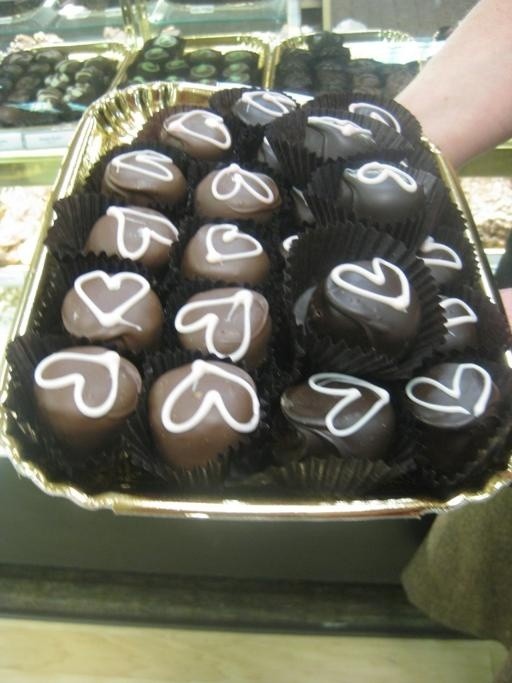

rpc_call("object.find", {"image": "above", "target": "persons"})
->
[392,0,512,353]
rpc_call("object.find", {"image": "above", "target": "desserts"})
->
[1,29,503,471]
